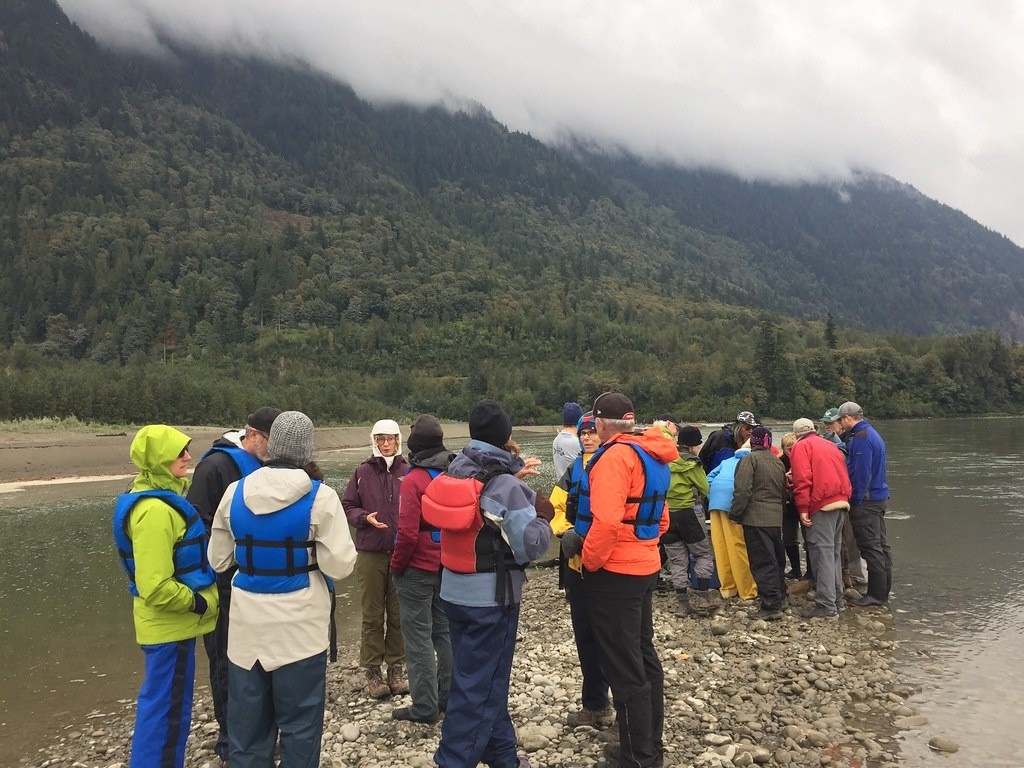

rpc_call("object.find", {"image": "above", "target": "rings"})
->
[802,520,807,524]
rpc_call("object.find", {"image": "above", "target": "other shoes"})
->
[660,562,896,621]
[593,742,663,768]
[393,707,439,724]
[516,757,530,768]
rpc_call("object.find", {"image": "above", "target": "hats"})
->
[593,392,635,420]
[735,411,760,426]
[793,418,816,435]
[407,414,444,452]
[819,407,841,423]
[830,401,863,421]
[749,428,772,448]
[676,426,703,447]
[562,402,583,425]
[657,413,685,428]
[267,411,314,466]
[578,423,596,432]
[246,405,283,434]
[469,398,512,447]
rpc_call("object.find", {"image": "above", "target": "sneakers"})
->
[365,664,412,697]
[567,706,616,727]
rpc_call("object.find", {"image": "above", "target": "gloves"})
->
[190,592,208,614]
[562,529,583,558]
[535,489,555,524]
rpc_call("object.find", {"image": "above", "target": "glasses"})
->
[178,446,188,459]
[375,435,396,445]
[741,425,756,432]
[578,430,597,436]
[822,421,839,426]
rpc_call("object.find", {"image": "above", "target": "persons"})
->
[434,397,556,768]
[184,406,286,761]
[390,413,458,725]
[654,401,893,620]
[343,418,411,699]
[581,391,679,768]
[551,401,584,600]
[204,410,358,768]
[115,424,220,768]
[549,410,621,731]
